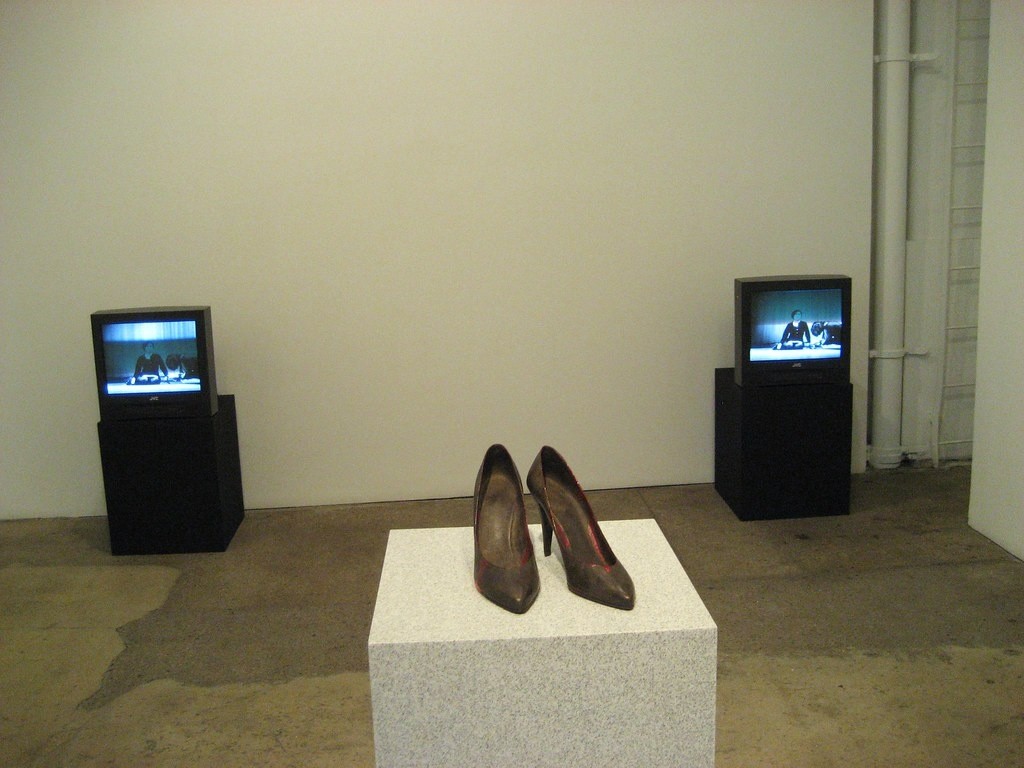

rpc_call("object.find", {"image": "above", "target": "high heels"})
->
[527,445,636,611]
[472,444,541,616]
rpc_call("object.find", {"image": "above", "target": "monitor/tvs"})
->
[90,306,218,421]
[735,274,853,386]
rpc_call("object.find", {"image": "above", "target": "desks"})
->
[96,393,244,557]
[713,367,853,522]
[366,518,719,768]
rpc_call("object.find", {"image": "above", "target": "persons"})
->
[127,342,200,385]
[773,310,842,350]
